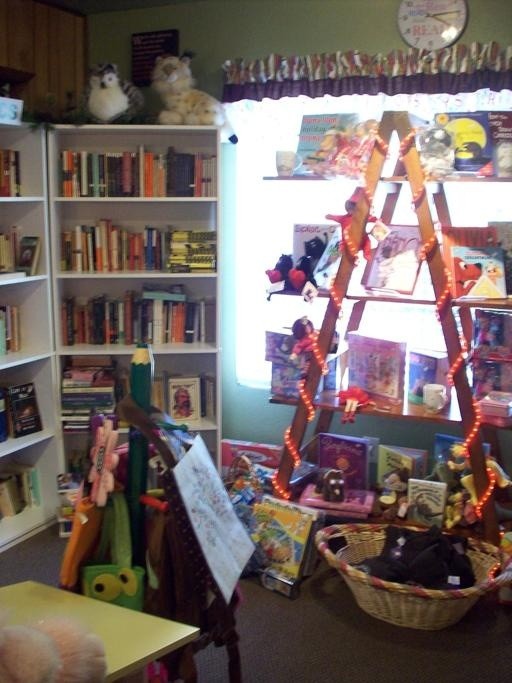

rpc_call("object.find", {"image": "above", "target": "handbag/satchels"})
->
[115,397,256,621]
[81,490,145,607]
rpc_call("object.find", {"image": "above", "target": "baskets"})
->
[315,522,512,633]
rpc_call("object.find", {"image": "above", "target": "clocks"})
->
[393,0,470,52]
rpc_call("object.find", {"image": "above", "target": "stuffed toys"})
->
[150,48,227,126]
[77,60,145,125]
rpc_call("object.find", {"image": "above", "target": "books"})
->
[220,108,512,583]
[55,143,217,538]
[1,149,43,516]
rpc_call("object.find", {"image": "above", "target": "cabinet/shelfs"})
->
[260,163,511,536]
